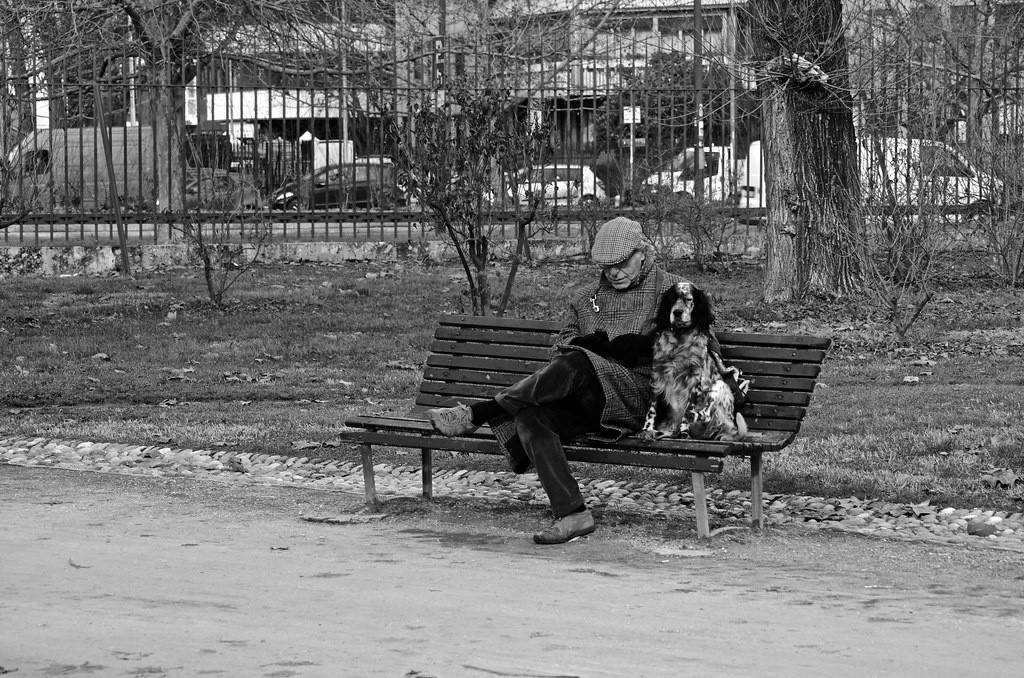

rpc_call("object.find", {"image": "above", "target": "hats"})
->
[591,217,643,266]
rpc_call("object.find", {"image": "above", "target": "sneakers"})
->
[428,401,480,437]
[533,508,596,544]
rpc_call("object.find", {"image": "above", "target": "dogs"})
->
[638,281,751,444]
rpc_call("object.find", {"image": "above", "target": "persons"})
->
[426,217,697,547]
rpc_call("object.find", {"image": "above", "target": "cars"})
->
[482,162,607,210]
[186,174,263,213]
[268,161,407,211]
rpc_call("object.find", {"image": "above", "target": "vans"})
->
[0,125,155,209]
[643,132,1005,209]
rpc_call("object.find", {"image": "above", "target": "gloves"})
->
[610,332,652,366]
[569,331,609,359]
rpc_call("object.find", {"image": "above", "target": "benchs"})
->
[339,313,832,542]
